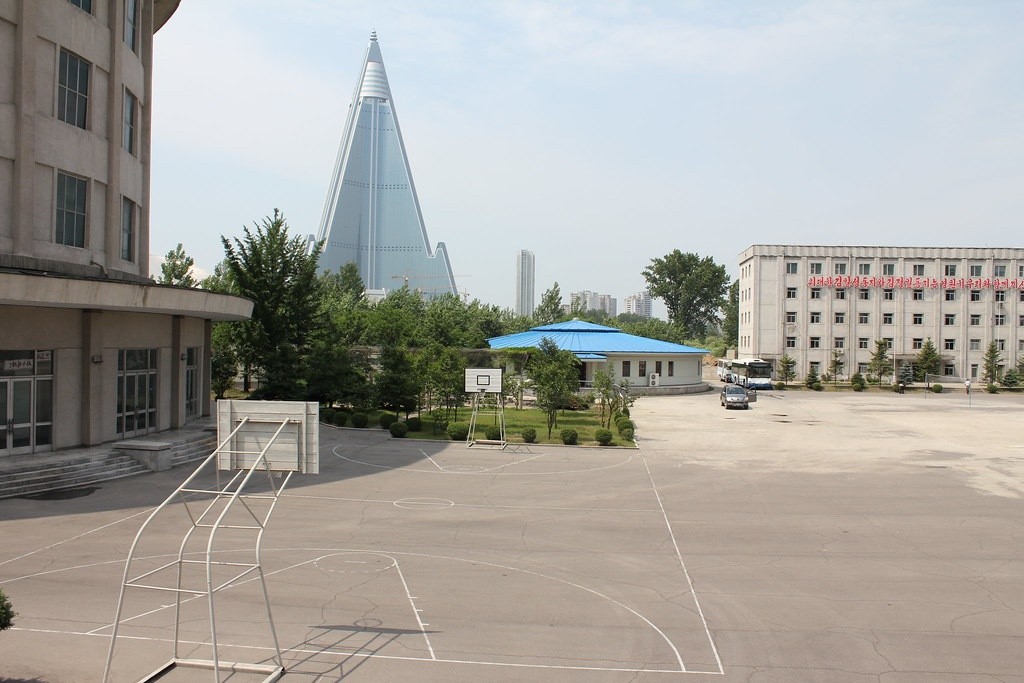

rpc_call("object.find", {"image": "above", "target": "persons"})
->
[898,383,904,394]
[965,379,971,394]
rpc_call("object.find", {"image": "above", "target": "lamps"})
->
[92,354,104,364]
[181,353,189,361]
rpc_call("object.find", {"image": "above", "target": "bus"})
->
[718,358,734,383]
[731,358,774,390]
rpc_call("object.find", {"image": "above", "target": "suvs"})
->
[720,384,757,410]
